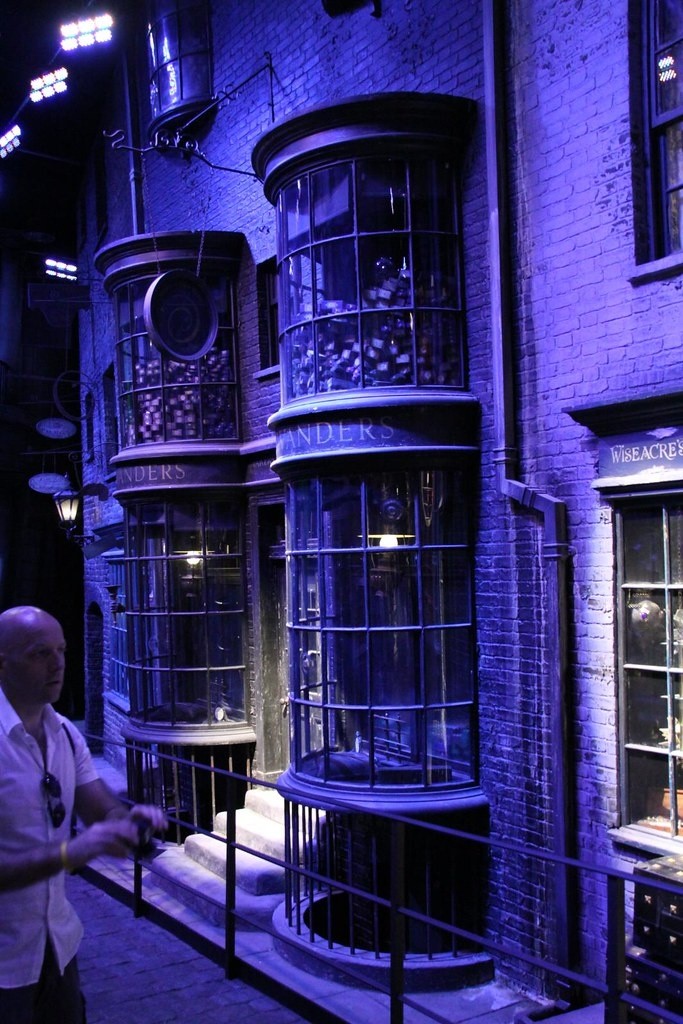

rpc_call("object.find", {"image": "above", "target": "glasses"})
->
[42,770,66,828]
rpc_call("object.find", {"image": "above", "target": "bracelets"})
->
[60,842,70,867]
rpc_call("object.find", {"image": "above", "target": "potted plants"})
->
[647,715,683,821]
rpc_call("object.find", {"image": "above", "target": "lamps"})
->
[173,534,215,596]
[357,473,415,596]
[55,475,95,546]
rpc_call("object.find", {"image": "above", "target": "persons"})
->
[0,606,169,1024]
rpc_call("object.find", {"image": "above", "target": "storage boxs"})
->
[623,927,683,1024]
[633,854,683,966]
